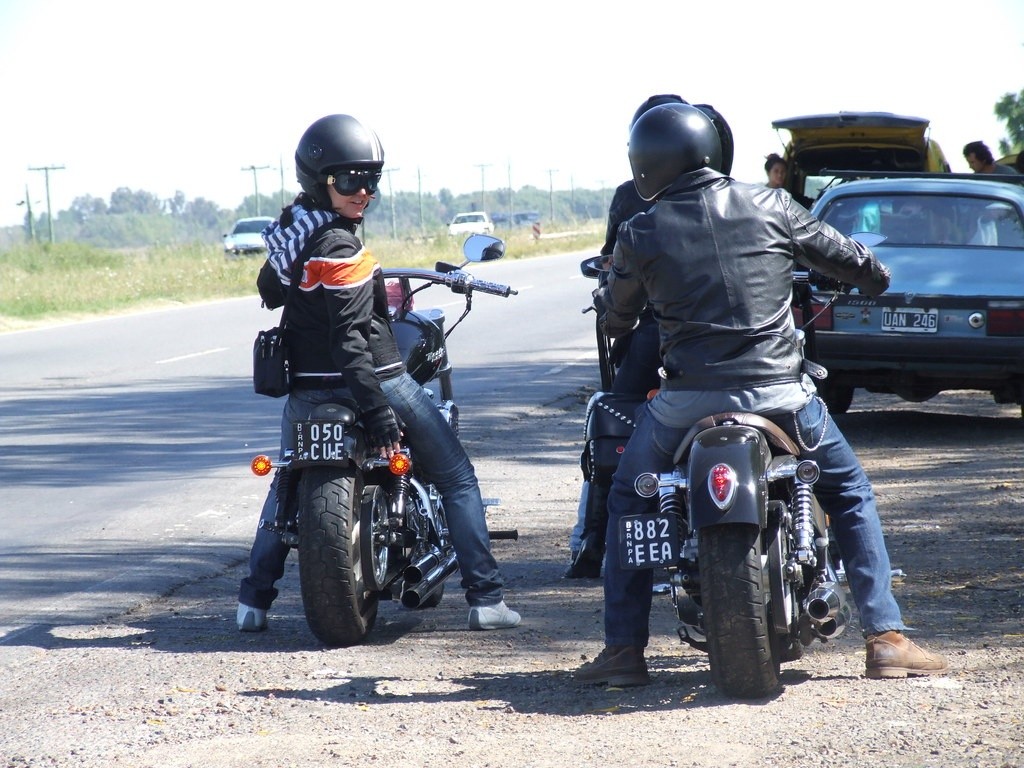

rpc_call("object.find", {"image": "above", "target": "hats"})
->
[629,94,692,135]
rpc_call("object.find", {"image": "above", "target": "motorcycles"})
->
[579,253,858,699]
[253,231,521,647]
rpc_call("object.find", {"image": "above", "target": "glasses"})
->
[331,171,382,197]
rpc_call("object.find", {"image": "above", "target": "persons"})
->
[236,115,522,632]
[565,92,947,688]
[963,141,1024,175]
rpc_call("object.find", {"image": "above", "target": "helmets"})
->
[294,113,383,211]
[691,104,734,176]
[627,103,723,202]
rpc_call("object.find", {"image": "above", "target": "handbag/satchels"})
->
[253,326,294,398]
[583,392,650,481]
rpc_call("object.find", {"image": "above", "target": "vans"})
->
[772,113,957,293]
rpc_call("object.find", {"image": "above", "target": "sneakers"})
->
[467,599,522,631]
[864,629,951,678]
[236,602,267,633]
[572,643,651,687]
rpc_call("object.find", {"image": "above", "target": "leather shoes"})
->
[563,560,602,579]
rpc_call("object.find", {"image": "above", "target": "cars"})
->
[793,170,1024,409]
[446,213,494,236]
[224,217,275,256]
